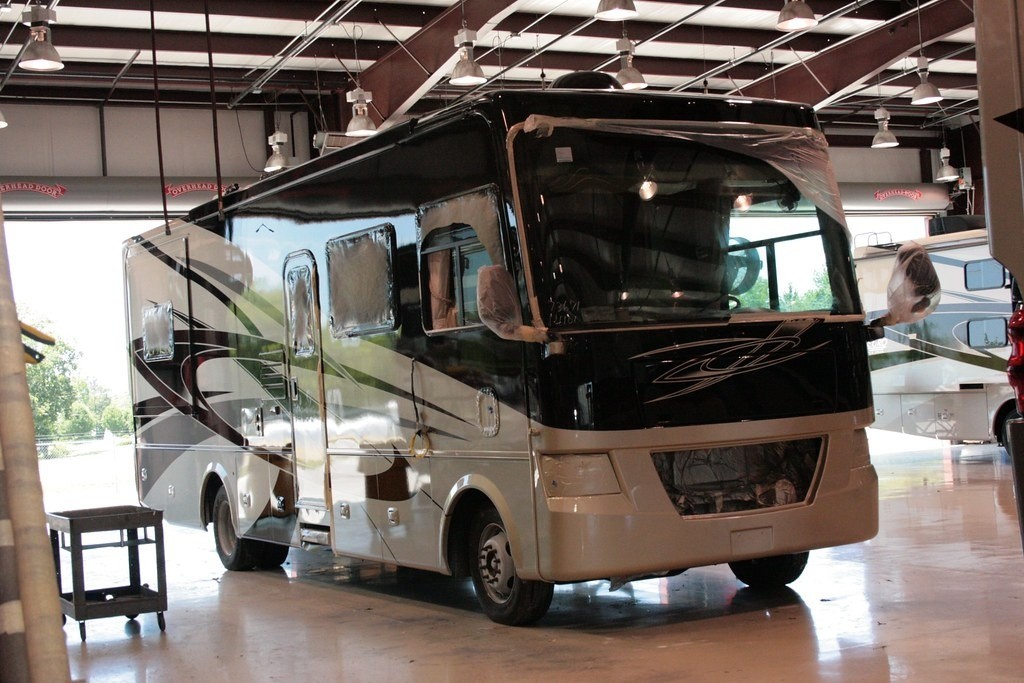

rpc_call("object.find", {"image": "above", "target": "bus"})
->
[118,70,943,627]
[850,213,1024,376]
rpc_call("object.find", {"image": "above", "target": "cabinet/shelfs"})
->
[47,506,168,642]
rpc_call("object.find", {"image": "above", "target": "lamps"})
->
[595,0,640,22]
[449,0,488,86]
[342,22,378,137]
[936,121,959,181]
[776,0,817,31]
[870,73,900,149]
[263,77,288,172]
[910,1,943,104]
[616,20,647,90]
[19,0,64,72]
